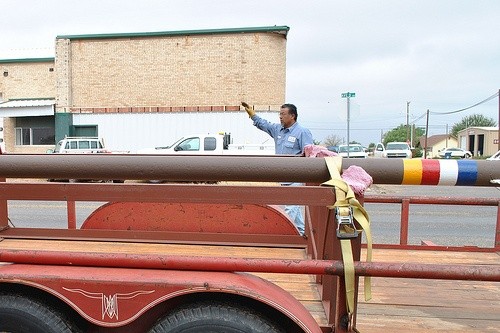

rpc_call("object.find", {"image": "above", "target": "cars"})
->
[436,146,473,160]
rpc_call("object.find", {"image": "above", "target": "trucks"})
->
[0,149,500,333]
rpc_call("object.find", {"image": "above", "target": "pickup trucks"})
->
[123,128,276,186]
[43,135,131,185]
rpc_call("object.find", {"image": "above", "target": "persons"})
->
[245,104,312,235]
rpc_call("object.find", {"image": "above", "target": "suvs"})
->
[334,143,370,159]
[374,141,413,159]
